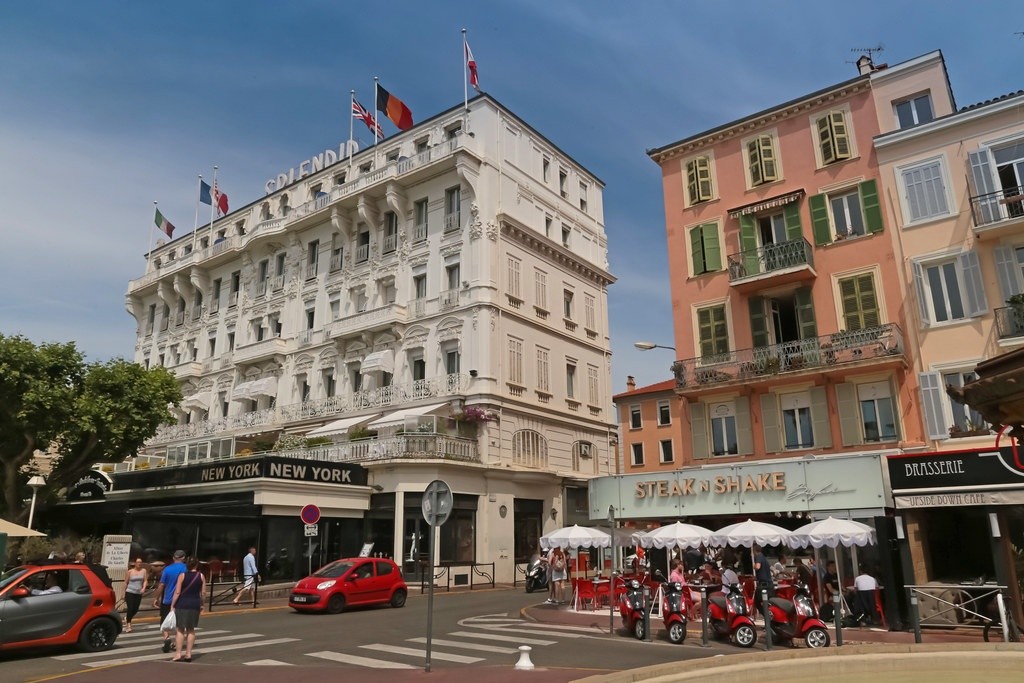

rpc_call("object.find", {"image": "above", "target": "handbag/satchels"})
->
[553,560,565,571]
[819,603,835,622]
[160,611,176,633]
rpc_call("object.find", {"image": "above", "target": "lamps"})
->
[634,342,675,351]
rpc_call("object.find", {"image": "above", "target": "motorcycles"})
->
[524,553,549,593]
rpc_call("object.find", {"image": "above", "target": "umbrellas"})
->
[540,516,877,607]
[0,519,47,536]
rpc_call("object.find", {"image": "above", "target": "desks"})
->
[198,561,231,578]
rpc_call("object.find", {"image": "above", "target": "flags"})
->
[466,39,483,96]
[154,170,229,239]
[351,83,415,139]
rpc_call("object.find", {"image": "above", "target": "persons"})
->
[542,546,879,626]
[76,548,262,662]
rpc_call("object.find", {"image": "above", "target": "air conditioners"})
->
[580,444,589,455]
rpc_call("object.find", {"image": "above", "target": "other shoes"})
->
[233,600,242,606]
[126,626,132,633]
[163,639,171,653]
[561,601,566,605]
[544,598,553,605]
[252,601,259,605]
[553,600,559,605]
[169,643,177,651]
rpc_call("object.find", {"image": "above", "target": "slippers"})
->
[171,657,183,662]
[181,656,191,662]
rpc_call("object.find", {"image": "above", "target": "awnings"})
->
[167,349,396,414]
[306,402,450,439]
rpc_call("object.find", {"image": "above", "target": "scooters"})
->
[707,568,758,648]
[760,584,830,649]
[654,569,693,644]
[611,571,651,641]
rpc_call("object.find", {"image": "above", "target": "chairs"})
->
[208,559,242,582]
[570,572,796,622]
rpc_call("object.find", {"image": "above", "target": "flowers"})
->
[463,407,487,422]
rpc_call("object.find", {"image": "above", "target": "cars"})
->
[0,559,122,654]
[288,557,408,615]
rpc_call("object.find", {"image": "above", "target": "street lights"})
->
[24,475,46,556]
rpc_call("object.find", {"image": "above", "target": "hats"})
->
[174,550,186,559]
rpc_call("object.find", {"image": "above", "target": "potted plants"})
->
[948,416,990,438]
